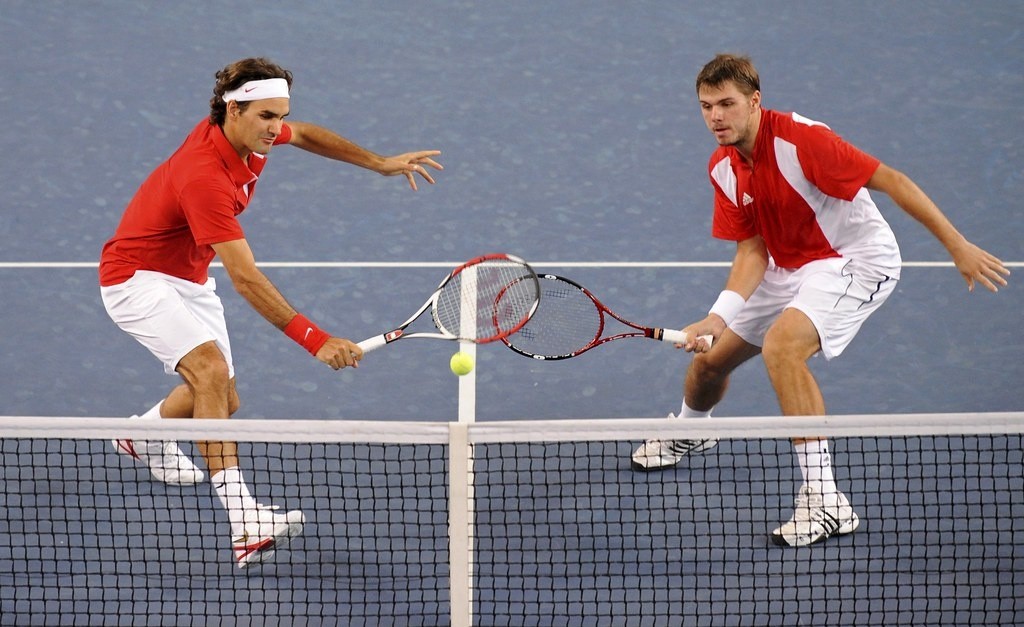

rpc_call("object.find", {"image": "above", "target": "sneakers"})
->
[770,486,860,547]
[231,505,306,568]
[111,415,205,486]
[630,413,720,472]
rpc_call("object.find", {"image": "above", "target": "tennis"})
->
[450,352,474,376]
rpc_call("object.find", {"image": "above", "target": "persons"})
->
[99,56,444,570]
[629,54,1009,547]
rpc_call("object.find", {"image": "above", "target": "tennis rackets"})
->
[490,273,714,361]
[326,253,542,368]
